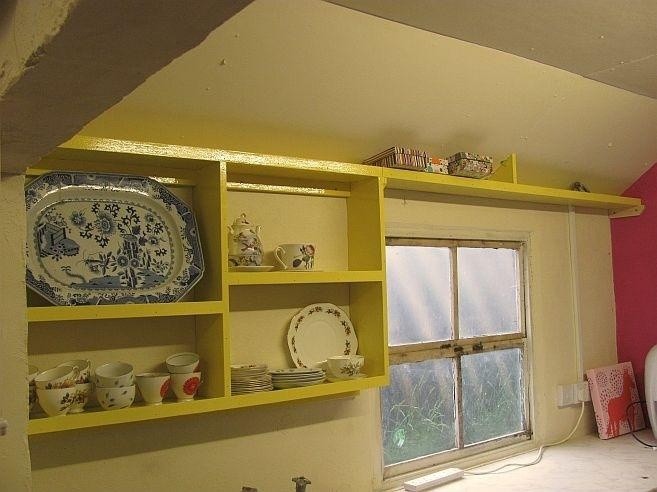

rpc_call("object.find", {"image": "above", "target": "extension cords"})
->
[403,467,464,492]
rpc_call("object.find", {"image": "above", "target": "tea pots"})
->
[228,213,264,266]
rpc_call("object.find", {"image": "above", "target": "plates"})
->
[227,265,273,272]
[277,267,323,271]
[25,172,204,308]
[231,301,358,394]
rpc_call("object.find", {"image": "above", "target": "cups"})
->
[275,242,316,270]
[27,351,204,417]
[328,355,364,379]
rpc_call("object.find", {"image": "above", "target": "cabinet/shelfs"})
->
[25,137,390,434]
